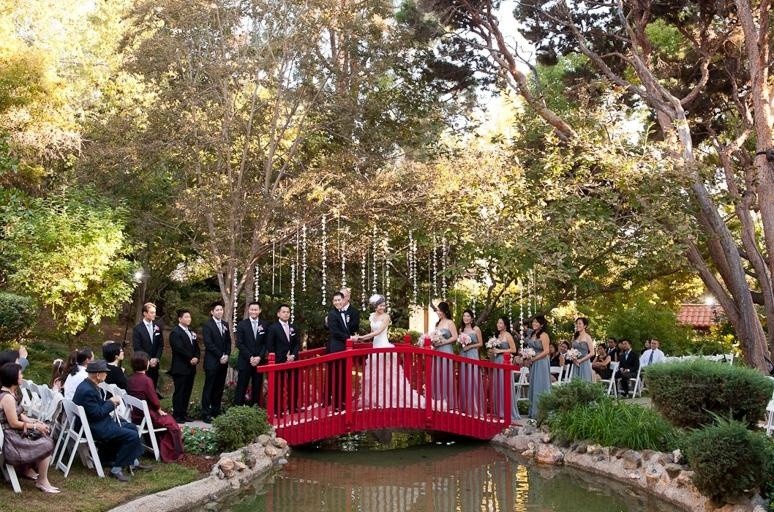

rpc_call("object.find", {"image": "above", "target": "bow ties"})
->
[339,309,346,314]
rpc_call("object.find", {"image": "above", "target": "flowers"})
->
[417,330,443,352]
[457,330,472,349]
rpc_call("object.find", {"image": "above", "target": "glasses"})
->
[120,349,125,352]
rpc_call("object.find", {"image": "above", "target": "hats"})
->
[84,359,113,374]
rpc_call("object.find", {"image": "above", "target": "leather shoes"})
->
[289,406,306,414]
[203,415,215,424]
[276,413,283,419]
[174,415,194,424]
[133,463,155,473]
[109,470,130,482]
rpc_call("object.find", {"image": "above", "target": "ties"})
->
[186,327,193,345]
[625,351,629,361]
[252,320,258,340]
[648,349,655,366]
[217,321,223,336]
[283,323,291,342]
[147,323,154,344]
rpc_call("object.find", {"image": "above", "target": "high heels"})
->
[35,482,61,494]
[23,471,41,481]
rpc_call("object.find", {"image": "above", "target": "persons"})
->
[328,292,358,412]
[458,309,486,418]
[357,294,446,411]
[487,317,522,418]
[325,288,359,408]
[513,316,666,419]
[425,301,458,411]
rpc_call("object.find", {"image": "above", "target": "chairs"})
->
[484,321,774,446]
[0,375,168,496]
